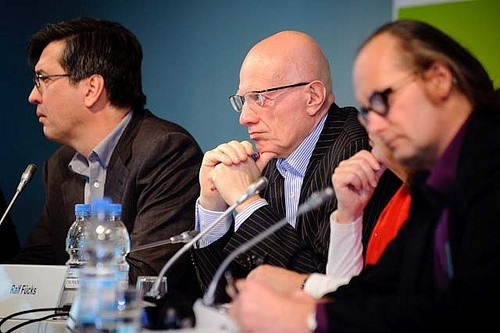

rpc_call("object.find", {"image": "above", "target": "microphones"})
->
[0,163,36,226]
[193,187,334,333]
[148,177,268,299]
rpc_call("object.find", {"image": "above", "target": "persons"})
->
[190,28,374,303]
[229,18,500,333]
[246,130,412,298]
[9,17,204,284]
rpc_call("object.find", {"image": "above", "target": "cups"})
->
[135,276,167,299]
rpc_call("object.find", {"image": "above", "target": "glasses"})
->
[228,82,311,113]
[32,72,72,88]
[355,67,425,125]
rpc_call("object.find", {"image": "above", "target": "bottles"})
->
[80,199,113,324]
[95,204,130,330]
[64,204,93,289]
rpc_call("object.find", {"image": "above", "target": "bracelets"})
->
[300,272,312,291]
[307,310,318,332]
